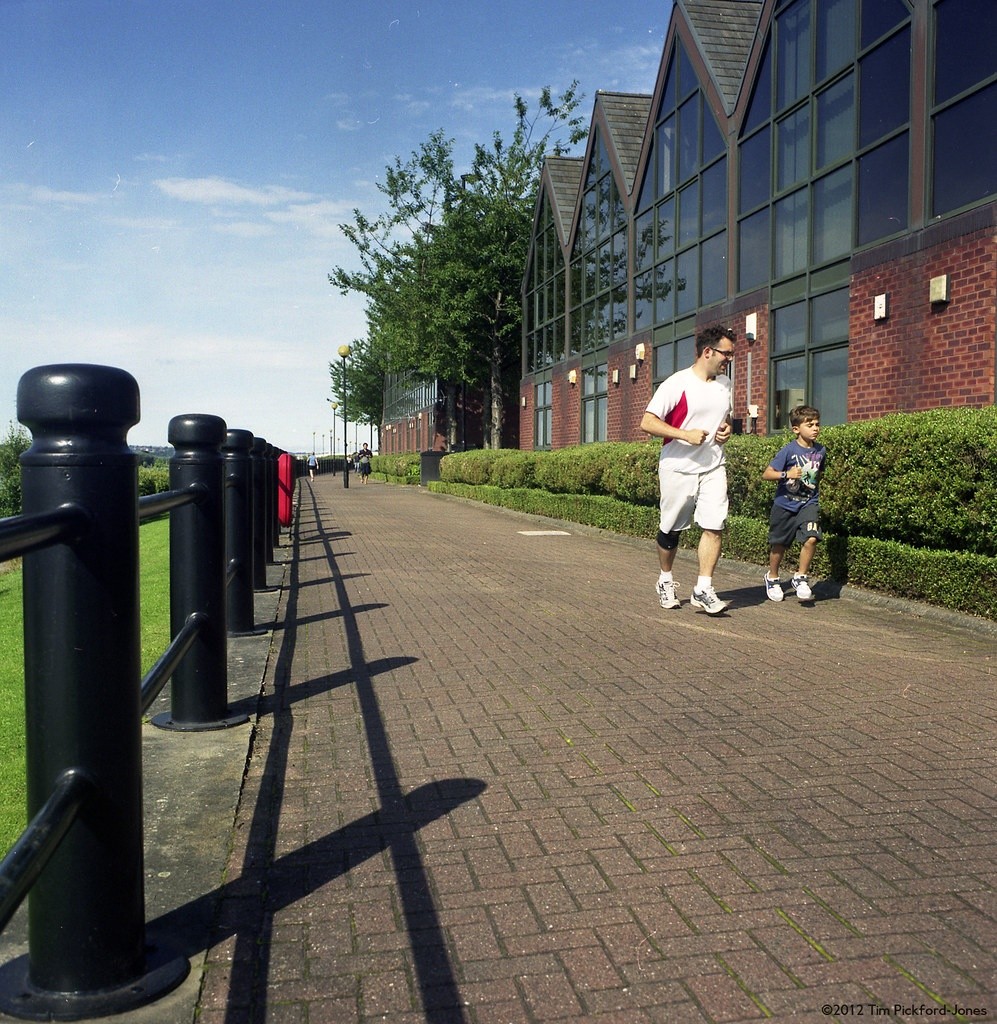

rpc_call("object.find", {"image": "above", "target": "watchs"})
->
[781,471,786,480]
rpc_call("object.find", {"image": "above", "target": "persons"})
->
[762,405,827,603]
[640,325,735,614]
[306,452,319,482]
[359,443,374,485]
[347,451,360,473]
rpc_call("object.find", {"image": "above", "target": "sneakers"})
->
[655,575,682,609]
[791,572,811,599]
[764,570,783,602]
[690,586,728,613]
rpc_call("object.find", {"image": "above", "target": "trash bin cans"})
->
[420,451,449,486]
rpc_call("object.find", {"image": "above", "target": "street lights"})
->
[330,403,337,476]
[337,344,352,488]
[312,429,362,455]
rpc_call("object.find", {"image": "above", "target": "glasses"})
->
[709,347,734,359]
